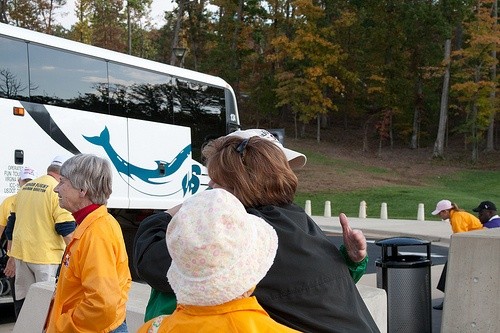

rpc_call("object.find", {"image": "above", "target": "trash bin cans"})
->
[374,238,434,333]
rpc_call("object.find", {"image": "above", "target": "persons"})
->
[145,129,368,323]
[133,136,381,333]
[433,200,500,310]
[5,157,76,283]
[135,188,303,333]
[46,154,132,333]
[0,166,36,322]
[431,199,484,232]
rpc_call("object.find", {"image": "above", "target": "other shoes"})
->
[433,302,443,310]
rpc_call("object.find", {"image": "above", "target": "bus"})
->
[0,22,240,209]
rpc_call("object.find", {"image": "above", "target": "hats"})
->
[166,188,279,307]
[473,201,497,212]
[431,199,455,215]
[51,155,68,167]
[226,128,307,169]
[19,168,36,180]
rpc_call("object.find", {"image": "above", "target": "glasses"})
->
[237,135,260,156]
